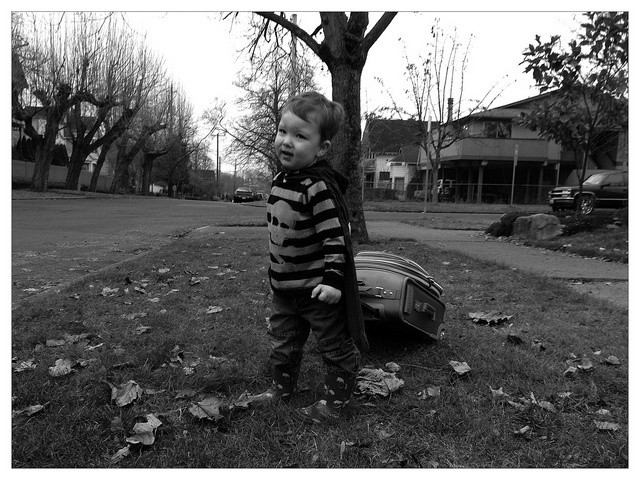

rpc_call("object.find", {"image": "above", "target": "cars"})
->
[235,187,253,200]
[550,171,627,212]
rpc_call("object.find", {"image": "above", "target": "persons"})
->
[254,91,362,424]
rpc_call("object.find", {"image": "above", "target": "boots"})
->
[291,377,356,426]
[246,368,297,408]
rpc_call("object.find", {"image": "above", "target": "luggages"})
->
[354,250,448,347]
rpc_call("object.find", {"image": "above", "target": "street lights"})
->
[510,141,519,204]
[422,116,433,215]
